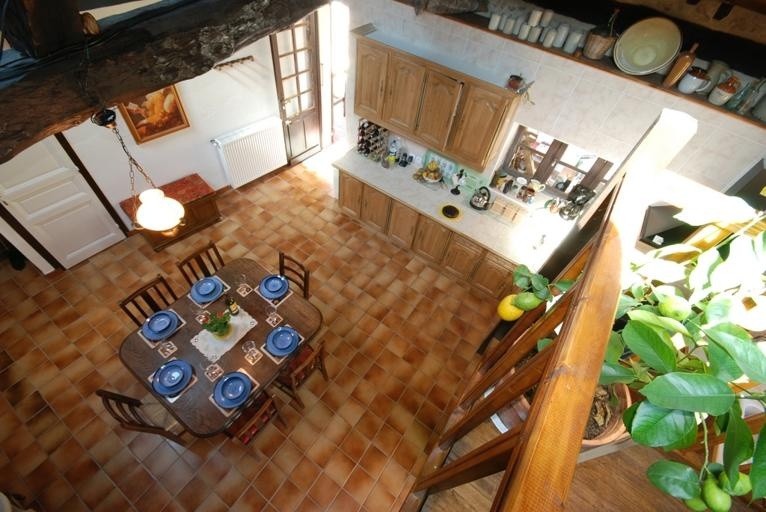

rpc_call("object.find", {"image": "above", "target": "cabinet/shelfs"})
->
[440,234,527,305]
[347,22,430,137]
[387,199,451,271]
[116,173,222,253]
[336,171,391,239]
[417,66,534,173]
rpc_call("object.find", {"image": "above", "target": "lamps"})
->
[89,103,188,240]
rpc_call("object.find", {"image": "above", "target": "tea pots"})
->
[469,186,494,212]
[528,178,546,193]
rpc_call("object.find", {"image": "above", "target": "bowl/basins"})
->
[159,364,183,387]
[272,329,292,348]
[221,376,246,401]
[264,277,283,292]
[148,313,171,333]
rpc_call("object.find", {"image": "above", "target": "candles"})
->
[486,5,583,54]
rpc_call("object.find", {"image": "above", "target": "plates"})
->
[151,359,193,396]
[422,172,442,183]
[190,277,223,303]
[614,16,682,77]
[258,274,287,299]
[142,310,178,340]
[194,278,217,295]
[213,371,251,407]
[266,326,298,356]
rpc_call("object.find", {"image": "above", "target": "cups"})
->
[487,7,582,55]
[509,76,523,89]
[678,65,711,95]
[188,302,210,323]
[199,358,217,375]
[516,186,535,204]
[265,304,278,324]
[150,334,167,354]
[242,340,257,360]
[516,176,527,188]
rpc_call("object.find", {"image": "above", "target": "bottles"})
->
[358,122,386,157]
[728,77,766,116]
[663,42,699,88]
[380,136,414,169]
[697,59,732,96]
[225,292,240,316]
[583,7,620,61]
[708,76,738,107]
[544,199,561,212]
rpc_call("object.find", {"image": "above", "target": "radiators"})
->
[211,116,286,191]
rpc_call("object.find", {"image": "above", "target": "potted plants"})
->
[491,232,765,512]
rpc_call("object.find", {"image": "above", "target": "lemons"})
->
[657,295,690,321]
[512,292,543,311]
[682,470,754,512]
[497,293,525,322]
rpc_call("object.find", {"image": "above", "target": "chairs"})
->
[223,386,286,450]
[114,273,177,330]
[176,239,224,289]
[270,335,330,410]
[93,385,191,447]
[274,249,310,302]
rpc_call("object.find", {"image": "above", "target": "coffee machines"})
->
[559,184,596,221]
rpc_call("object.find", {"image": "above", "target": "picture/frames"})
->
[117,83,190,145]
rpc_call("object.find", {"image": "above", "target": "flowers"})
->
[201,308,231,338]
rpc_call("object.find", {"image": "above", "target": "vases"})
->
[213,324,234,341]
[507,73,523,91]
